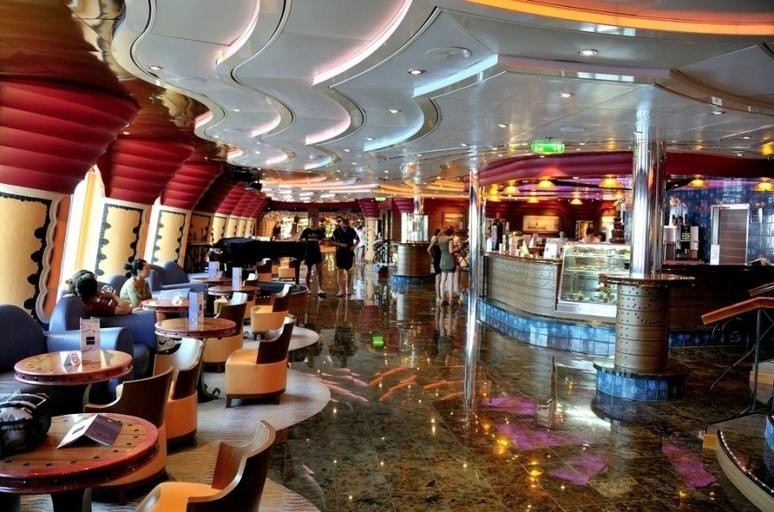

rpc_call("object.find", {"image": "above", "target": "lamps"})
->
[753,177,774,193]
[486,174,621,206]
[688,174,710,188]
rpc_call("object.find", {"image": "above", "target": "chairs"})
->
[241,260,310,327]
[136,420,277,511]
[224,318,297,408]
[1,260,241,503]
[248,283,293,340]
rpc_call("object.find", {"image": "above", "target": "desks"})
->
[1,412,160,511]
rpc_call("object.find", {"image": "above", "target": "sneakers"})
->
[336,287,353,297]
[317,290,328,295]
[436,297,453,306]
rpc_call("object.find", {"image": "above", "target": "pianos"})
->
[217,237,303,285]
[189,240,213,247]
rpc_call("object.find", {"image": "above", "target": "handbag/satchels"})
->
[0,392,53,461]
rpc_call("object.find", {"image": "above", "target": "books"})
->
[54,413,122,449]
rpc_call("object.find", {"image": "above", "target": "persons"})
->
[350,262,367,301]
[271,215,300,240]
[296,213,328,295]
[350,218,367,262]
[328,296,358,367]
[328,215,360,297]
[671,214,683,227]
[426,226,491,305]
[319,214,341,240]
[118,258,166,322]
[301,294,324,367]
[424,306,459,368]
[580,227,600,243]
[67,269,131,316]
